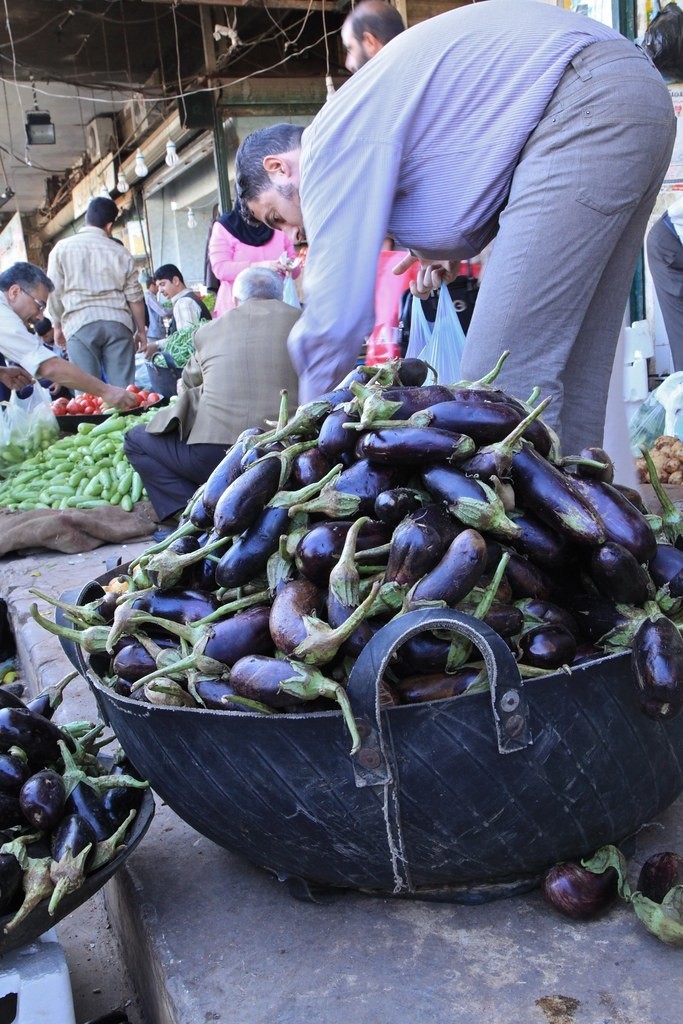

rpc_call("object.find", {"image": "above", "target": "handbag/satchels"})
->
[397,275,475,358]
[0,379,61,476]
[283,270,301,309]
[406,278,465,386]
[635,0,677,83]
[630,372,683,454]
[643,2,683,80]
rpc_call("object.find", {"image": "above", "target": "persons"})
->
[207,199,304,316]
[0,261,211,413]
[338,1,406,253]
[120,266,301,543]
[45,198,148,397]
[638,1,683,380]
[234,0,676,459]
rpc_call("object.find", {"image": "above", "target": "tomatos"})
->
[48,384,160,416]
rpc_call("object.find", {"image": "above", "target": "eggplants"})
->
[545,844,683,948]
[0,590,175,945]
[72,350,682,723]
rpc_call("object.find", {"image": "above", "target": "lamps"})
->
[23,72,58,146]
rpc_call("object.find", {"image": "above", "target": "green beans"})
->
[150,317,212,368]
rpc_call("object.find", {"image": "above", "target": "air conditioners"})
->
[85,117,116,164]
[123,88,165,138]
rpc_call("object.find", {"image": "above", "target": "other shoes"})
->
[152,527,177,543]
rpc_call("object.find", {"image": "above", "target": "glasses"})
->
[21,288,44,310]
